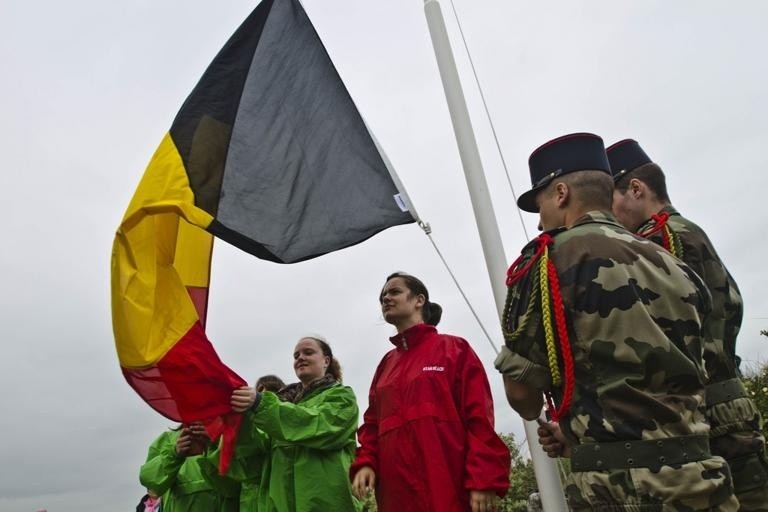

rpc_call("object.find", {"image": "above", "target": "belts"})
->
[706,376,750,408]
[570,433,712,472]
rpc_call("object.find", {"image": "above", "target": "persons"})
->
[608,138,767,512]
[233,336,360,511]
[138,416,242,512]
[214,375,289,511]
[136,487,161,512]
[346,272,513,512]
[496,131,743,511]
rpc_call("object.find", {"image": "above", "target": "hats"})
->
[605,139,652,182]
[517,132,613,213]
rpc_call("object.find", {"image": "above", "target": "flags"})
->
[93,0,413,472]
